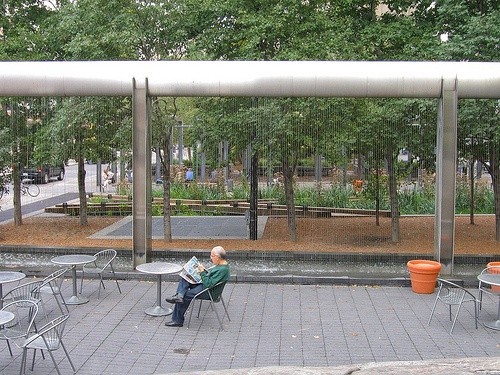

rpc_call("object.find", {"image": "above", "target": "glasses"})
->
[210,255,215,259]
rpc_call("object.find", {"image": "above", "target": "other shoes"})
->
[165,320,183,327]
[166,293,183,303]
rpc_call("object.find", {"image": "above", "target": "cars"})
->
[19,158,66,184]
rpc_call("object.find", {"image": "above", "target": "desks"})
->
[136,262,184,317]
[477,274,500,331]
[51,255,96,305]
[0,271,26,329]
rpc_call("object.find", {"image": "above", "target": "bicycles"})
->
[20,183,40,198]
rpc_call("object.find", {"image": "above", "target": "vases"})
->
[487,262,500,292]
[407,260,441,294]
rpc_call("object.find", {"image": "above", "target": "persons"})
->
[102,166,114,188]
[165,246,230,327]
[186,168,193,186]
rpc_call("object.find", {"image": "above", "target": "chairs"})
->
[428,266,500,335]
[187,280,231,331]
[79,249,121,300]
[0,268,77,375]
